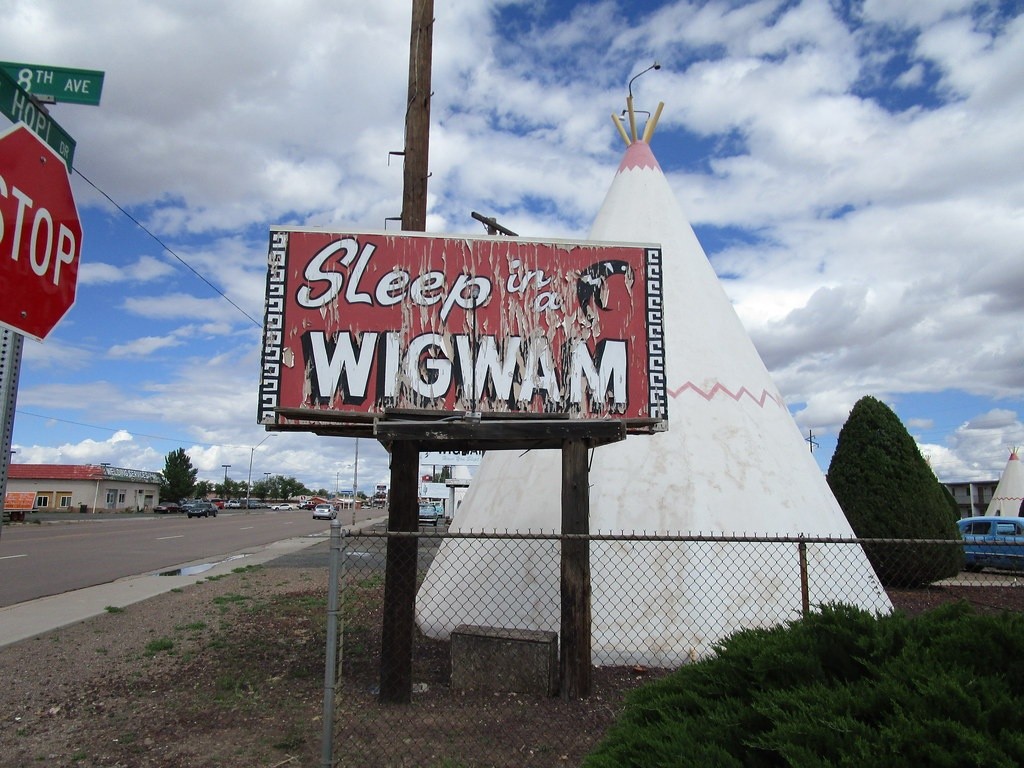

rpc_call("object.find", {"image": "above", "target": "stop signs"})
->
[1,122,85,340]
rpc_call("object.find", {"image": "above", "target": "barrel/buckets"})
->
[80,505,87,513]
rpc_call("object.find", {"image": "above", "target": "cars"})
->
[958,516,1023,574]
[153,503,181,515]
[419,504,440,527]
[242,500,262,509]
[312,504,338,520]
[261,503,294,511]
[297,501,317,509]
[187,503,219,519]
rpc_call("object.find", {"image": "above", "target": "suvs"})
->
[181,499,203,513]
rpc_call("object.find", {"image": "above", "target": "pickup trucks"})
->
[223,500,242,510]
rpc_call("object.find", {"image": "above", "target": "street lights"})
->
[336,465,351,498]
[246,434,277,514]
